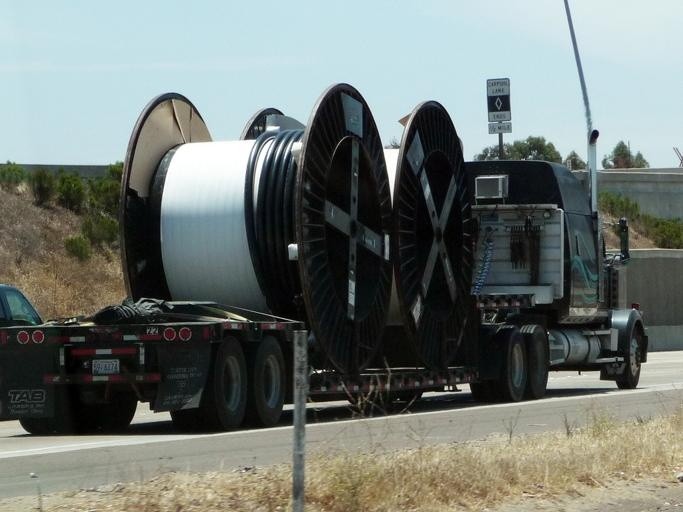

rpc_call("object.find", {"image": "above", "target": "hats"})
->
[0,285,44,329]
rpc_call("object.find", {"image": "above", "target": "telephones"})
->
[487,122,512,135]
[486,77,511,121]
